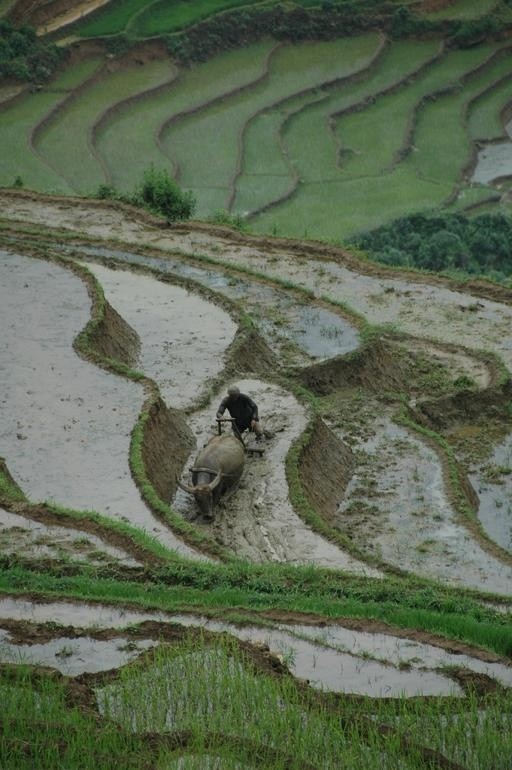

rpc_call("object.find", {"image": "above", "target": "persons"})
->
[216,385,262,439]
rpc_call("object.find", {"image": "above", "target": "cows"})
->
[173,431,244,524]
